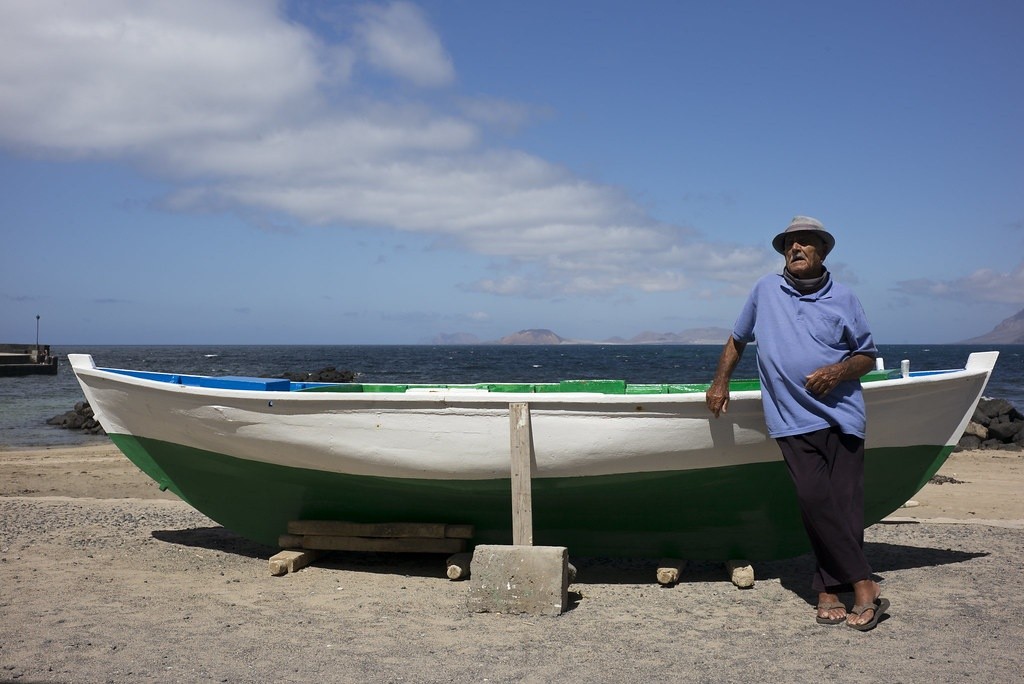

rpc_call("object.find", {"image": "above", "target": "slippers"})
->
[846,598,890,631]
[816,602,846,624]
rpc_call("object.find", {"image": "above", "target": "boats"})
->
[68,351,1000,559]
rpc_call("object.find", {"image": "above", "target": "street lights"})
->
[36,314,40,351]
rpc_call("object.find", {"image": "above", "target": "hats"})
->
[772,215,836,257]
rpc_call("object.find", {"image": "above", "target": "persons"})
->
[706,215,890,631]
